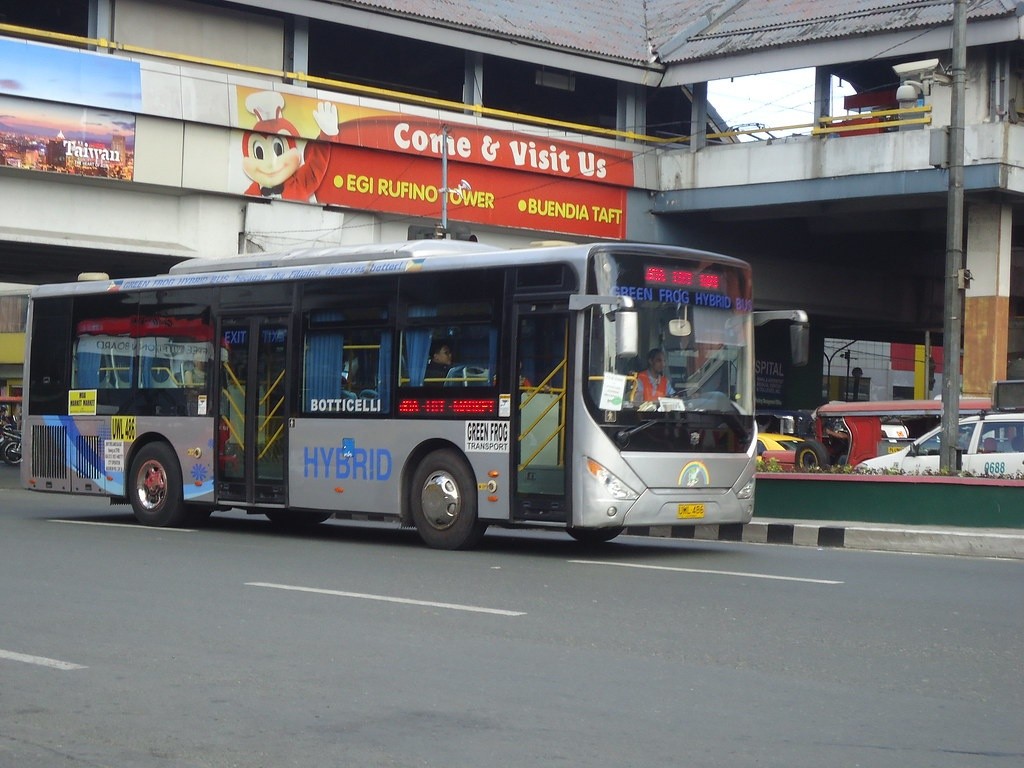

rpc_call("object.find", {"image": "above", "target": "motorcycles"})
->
[0,423,22,465]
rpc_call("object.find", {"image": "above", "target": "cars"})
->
[757,433,805,471]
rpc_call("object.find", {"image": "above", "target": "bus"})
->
[810,398,992,468]
[20,239,810,552]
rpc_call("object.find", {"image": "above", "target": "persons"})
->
[821,422,850,466]
[425,342,456,388]
[185,350,206,387]
[633,348,679,406]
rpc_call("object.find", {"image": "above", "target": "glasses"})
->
[444,351,450,355]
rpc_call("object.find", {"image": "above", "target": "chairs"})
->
[340,389,379,411]
[443,365,488,387]
[180,360,197,389]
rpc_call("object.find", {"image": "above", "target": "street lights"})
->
[892,58,975,476]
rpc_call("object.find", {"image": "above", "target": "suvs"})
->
[854,407,1024,479]
